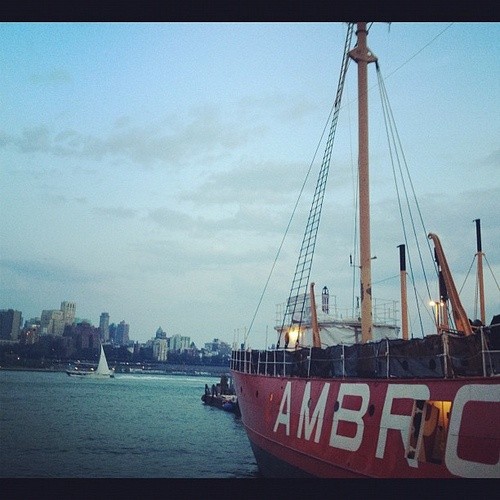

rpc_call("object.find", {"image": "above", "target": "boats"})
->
[202,374,239,412]
[65,362,114,377]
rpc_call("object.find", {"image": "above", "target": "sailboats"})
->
[96,345,110,378]
[227,23,499,477]
